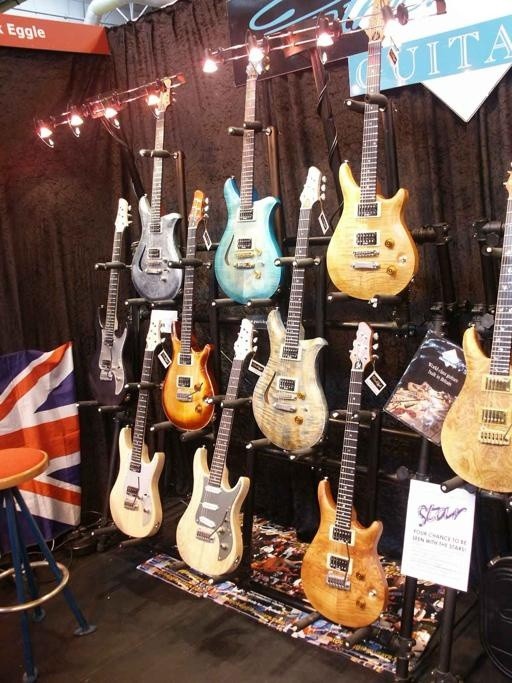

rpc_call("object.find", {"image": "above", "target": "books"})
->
[381,329,468,449]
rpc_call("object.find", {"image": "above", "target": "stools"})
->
[0,447,98,683]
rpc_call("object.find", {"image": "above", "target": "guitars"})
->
[129,77,183,304]
[110,319,166,542]
[252,165,329,458]
[325,0,422,302]
[213,30,284,303]
[175,316,259,579]
[87,198,133,407]
[299,319,391,631]
[440,165,512,493]
[162,190,216,433]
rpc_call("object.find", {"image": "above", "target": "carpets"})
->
[135,510,447,676]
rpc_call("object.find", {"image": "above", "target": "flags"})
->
[1,340,81,555]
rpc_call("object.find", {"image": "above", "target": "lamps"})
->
[32,72,186,148]
[202,0,447,74]
